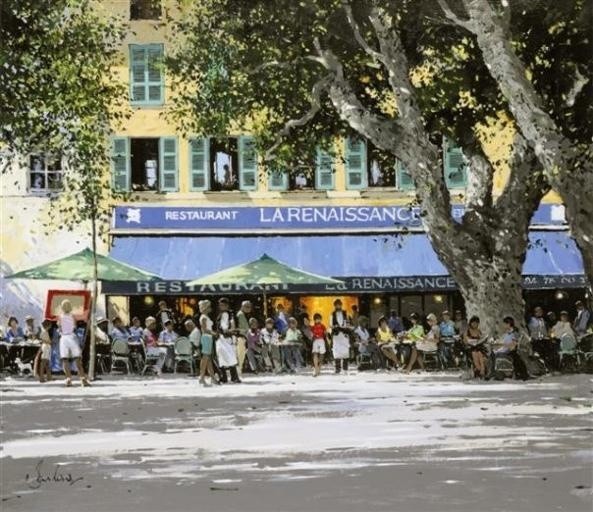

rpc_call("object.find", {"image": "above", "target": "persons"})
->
[222,162,231,183]
[31,157,62,189]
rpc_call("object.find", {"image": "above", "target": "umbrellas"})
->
[184,253,346,319]
[1,246,163,312]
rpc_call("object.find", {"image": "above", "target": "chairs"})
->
[0,323,593,381]
